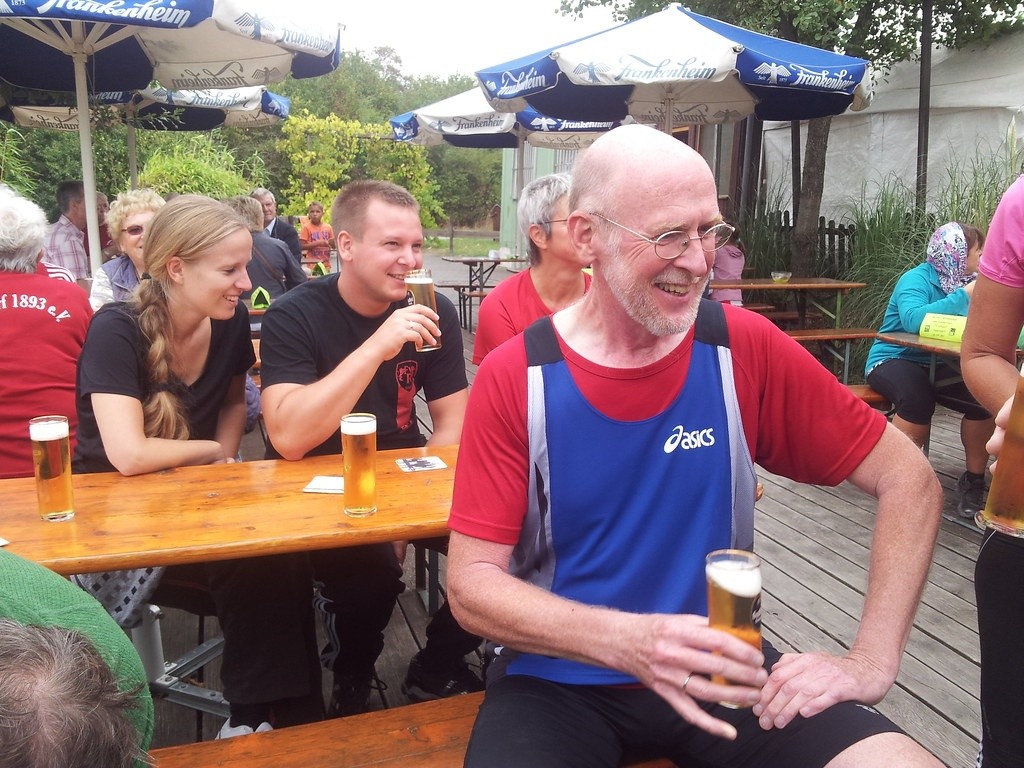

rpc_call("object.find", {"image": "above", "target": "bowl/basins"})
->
[771,270,791,283]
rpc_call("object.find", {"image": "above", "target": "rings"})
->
[682,673,693,689]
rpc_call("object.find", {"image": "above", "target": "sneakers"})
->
[401,647,487,703]
[956,471,988,519]
[328,668,375,717]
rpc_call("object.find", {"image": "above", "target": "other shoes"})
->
[212,715,274,740]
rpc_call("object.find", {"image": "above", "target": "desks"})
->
[877,333,1024,458]
[709,277,866,375]
[442,256,530,333]
[301,259,323,264]
[0,445,459,576]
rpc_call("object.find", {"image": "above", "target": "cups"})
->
[982,361,1024,540]
[29,413,74,522]
[403,268,442,352]
[706,551,764,710]
[340,413,377,518]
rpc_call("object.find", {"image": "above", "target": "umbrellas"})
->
[474,3,873,136]
[0,0,346,279]
[389,86,656,273]
[0,85,292,193]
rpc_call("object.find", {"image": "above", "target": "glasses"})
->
[121,225,145,236]
[589,211,738,259]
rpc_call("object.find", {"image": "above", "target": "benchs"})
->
[740,302,892,411]
[434,283,496,330]
[147,691,676,768]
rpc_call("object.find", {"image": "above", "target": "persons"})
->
[0,620,140,768]
[0,197,94,479]
[259,180,486,716]
[446,124,945,768]
[959,174,1024,768]
[71,195,328,740]
[0,181,336,462]
[710,221,745,306]
[472,173,592,366]
[865,221,995,519]
